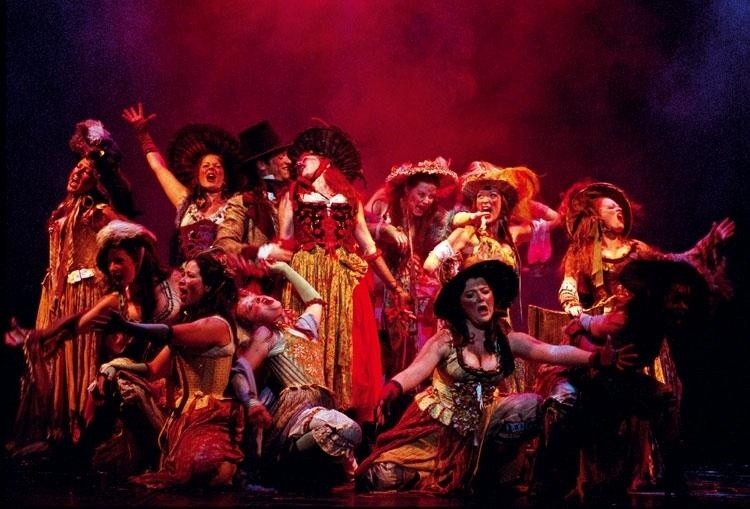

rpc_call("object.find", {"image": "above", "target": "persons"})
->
[273,126,410,416]
[419,159,560,333]
[215,117,302,265]
[354,156,492,333]
[552,178,737,335]
[122,97,251,257]
[1,217,749,508]
[24,148,127,436]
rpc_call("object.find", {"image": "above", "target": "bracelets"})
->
[389,281,399,293]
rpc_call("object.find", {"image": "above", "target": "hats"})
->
[94,235,153,276]
[433,253,522,321]
[462,161,520,208]
[235,120,294,163]
[386,161,460,189]
[288,125,362,184]
[166,124,241,192]
[565,182,634,240]
[76,123,139,220]
